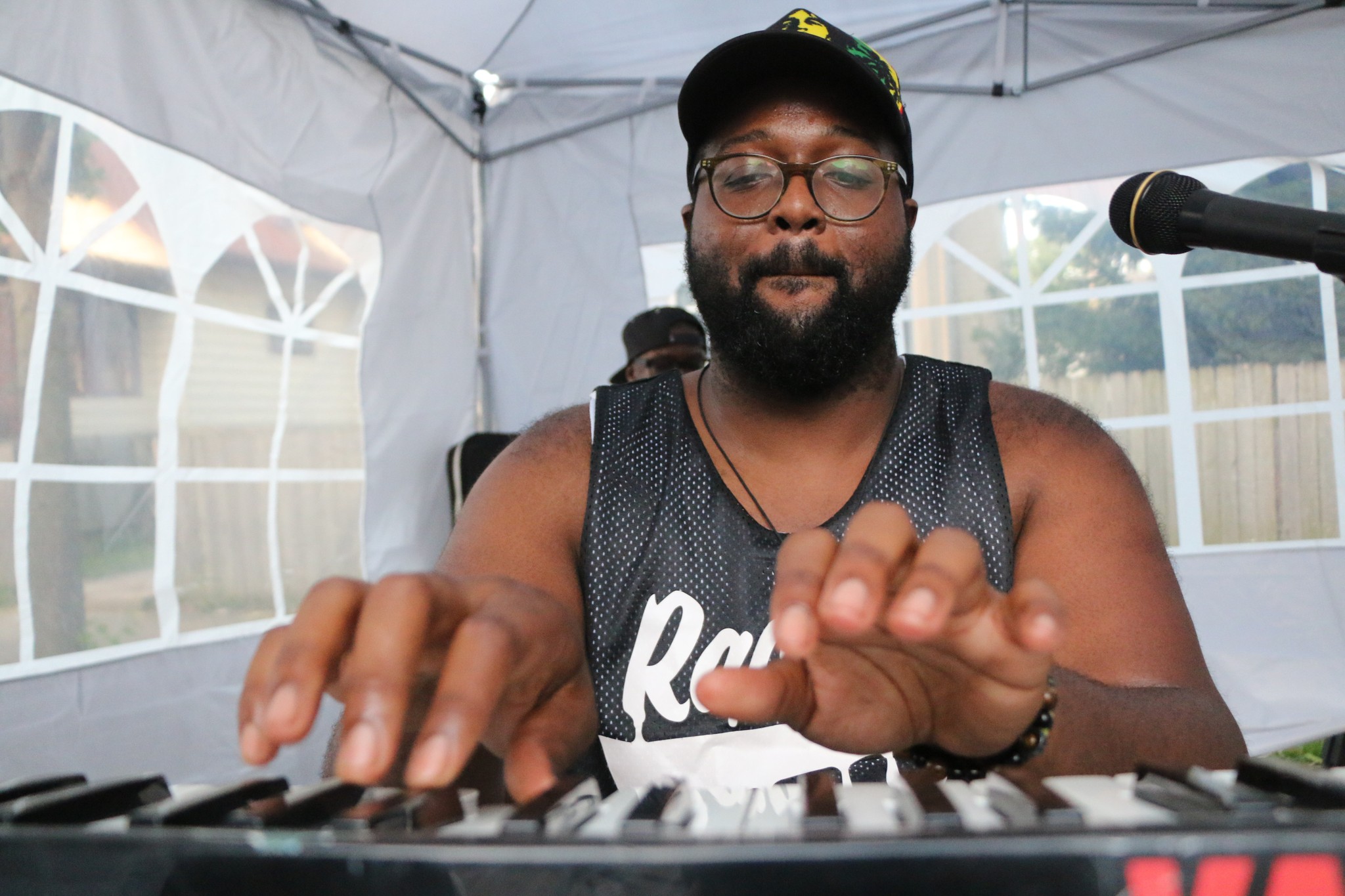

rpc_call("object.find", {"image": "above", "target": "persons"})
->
[234,6,1250,817]
[608,304,707,389]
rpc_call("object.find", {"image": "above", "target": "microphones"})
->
[1108,169,1345,279]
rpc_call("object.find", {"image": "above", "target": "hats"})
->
[610,307,706,384]
[676,9,913,191]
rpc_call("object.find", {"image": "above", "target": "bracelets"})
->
[895,670,1063,783]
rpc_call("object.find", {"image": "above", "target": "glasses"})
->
[633,356,709,370]
[691,153,909,222]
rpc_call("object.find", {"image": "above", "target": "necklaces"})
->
[695,363,908,544]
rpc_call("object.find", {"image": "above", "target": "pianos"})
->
[0,746,1345,896]
